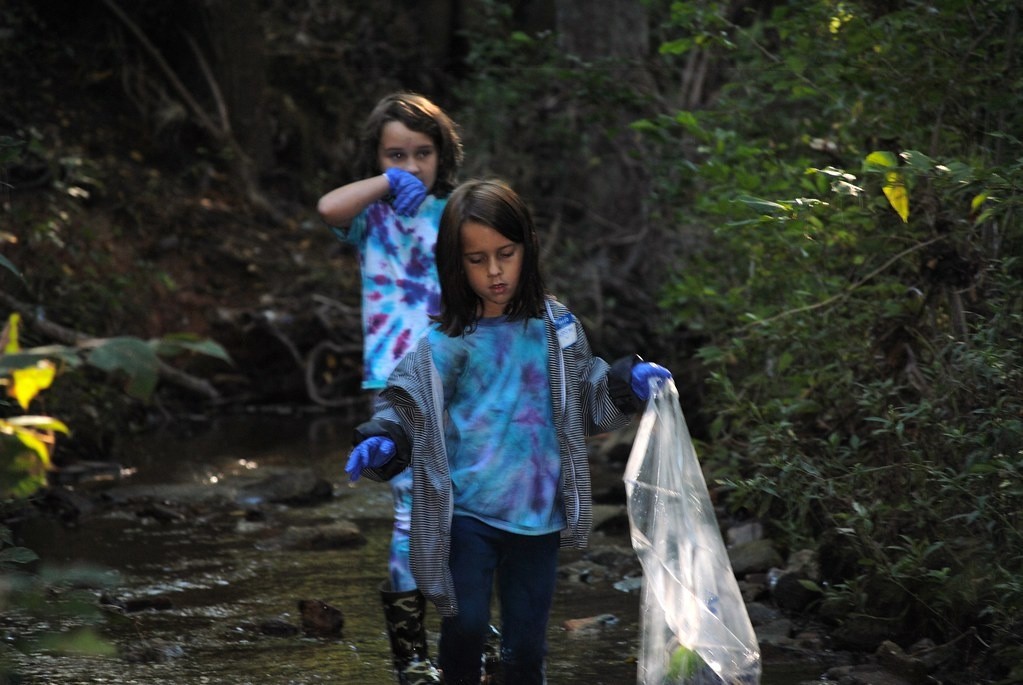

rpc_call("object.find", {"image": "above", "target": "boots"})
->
[378,578,443,685]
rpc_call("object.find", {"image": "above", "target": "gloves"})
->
[629,359,675,403]
[383,165,427,219]
[345,435,397,482]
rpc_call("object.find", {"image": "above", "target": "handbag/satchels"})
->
[622,373,762,685]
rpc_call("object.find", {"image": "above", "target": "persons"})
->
[342,170,675,681]
[315,85,504,683]
